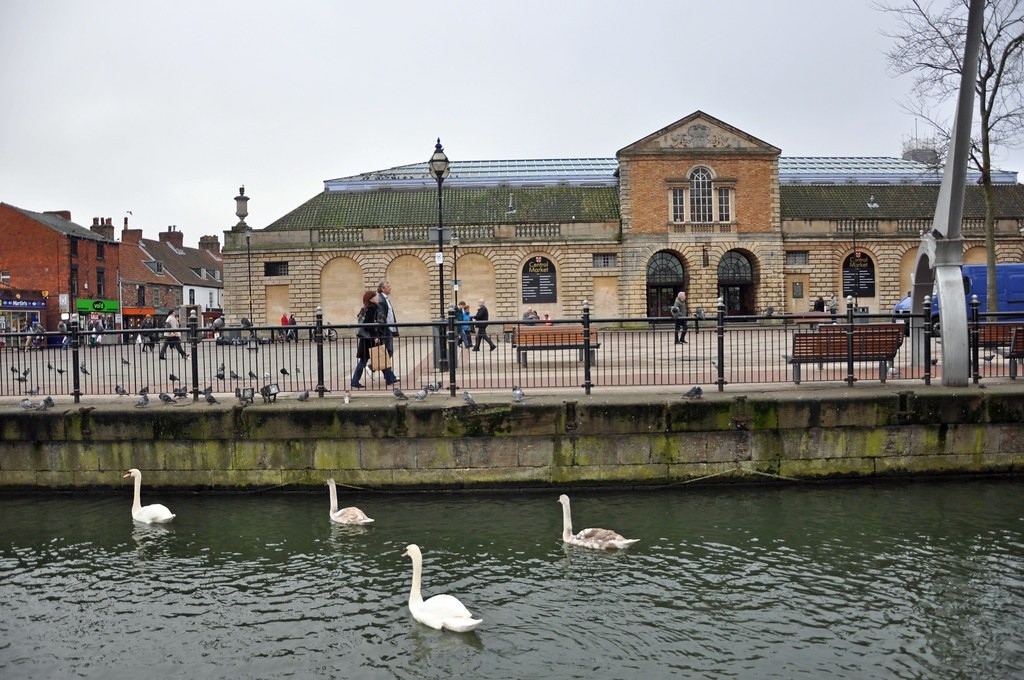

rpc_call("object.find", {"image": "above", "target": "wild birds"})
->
[209,314,253,333]
[297,385,351,404]
[32,320,69,375]
[20,395,55,412]
[159,374,221,406]
[392,387,409,400]
[117,385,150,408]
[463,392,475,406]
[80,360,91,375]
[512,386,524,402]
[415,381,443,401]
[10,366,40,396]
[248,367,302,379]
[122,357,132,365]
[680,337,997,402]
[213,363,242,380]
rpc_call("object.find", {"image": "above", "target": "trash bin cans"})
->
[854,306,870,324]
[229,324,241,339]
[433,322,459,368]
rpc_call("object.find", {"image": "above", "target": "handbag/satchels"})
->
[355,326,372,339]
[369,340,392,371]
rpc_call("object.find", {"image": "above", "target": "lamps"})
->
[238,387,254,404]
[168,285,173,293]
[260,384,280,404]
[82,280,88,293]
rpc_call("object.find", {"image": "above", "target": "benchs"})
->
[814,319,907,367]
[1000,329,1024,379]
[965,321,1024,378]
[784,328,900,383]
[504,319,553,335]
[513,323,598,366]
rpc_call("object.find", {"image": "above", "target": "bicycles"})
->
[311,321,338,343]
[276,329,296,344]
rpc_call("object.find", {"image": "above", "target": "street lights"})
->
[245,226,261,349]
[428,137,451,373]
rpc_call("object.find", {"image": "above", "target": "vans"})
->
[892,263,1024,338]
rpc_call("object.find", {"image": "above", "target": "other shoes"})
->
[183,353,190,358]
[158,355,166,360]
[489,345,496,352]
[388,377,401,384]
[351,382,366,388]
[472,347,478,351]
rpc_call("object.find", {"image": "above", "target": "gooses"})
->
[557,494,641,550]
[124,468,175,525]
[401,544,483,633]
[327,478,375,527]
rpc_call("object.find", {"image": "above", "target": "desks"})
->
[789,311,833,335]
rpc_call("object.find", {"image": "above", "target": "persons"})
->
[280,313,296,341]
[88,315,114,349]
[673,291,689,344]
[829,292,837,323]
[159,310,190,360]
[140,314,155,352]
[454,301,474,348]
[811,296,824,312]
[470,300,497,351]
[523,307,540,320]
[351,280,400,388]
[544,314,553,326]
[19,319,46,352]
[62,322,72,350]
